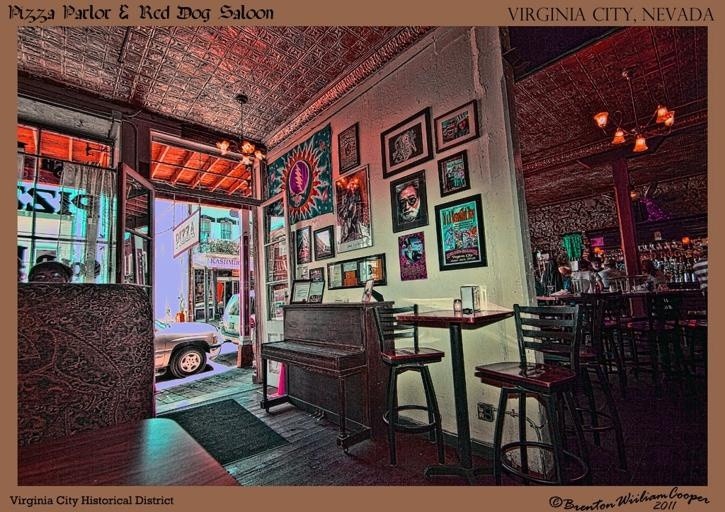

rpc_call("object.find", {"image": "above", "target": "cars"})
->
[153,317,221,378]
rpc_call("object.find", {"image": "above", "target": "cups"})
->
[547,275,660,296]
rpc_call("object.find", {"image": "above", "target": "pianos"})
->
[258,302,398,455]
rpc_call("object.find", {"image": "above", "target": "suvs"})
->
[222,291,255,348]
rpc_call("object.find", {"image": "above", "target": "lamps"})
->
[594,68,675,153]
[216,92,268,166]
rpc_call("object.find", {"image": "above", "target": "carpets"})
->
[157,399,291,466]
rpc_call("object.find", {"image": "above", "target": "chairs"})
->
[539,285,708,466]
[473,303,593,487]
[373,305,446,466]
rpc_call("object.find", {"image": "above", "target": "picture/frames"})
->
[289,98,489,303]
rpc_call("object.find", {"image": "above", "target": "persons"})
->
[28,261,73,283]
[692,245,708,297]
[396,180,422,226]
[540,253,626,353]
[641,260,665,281]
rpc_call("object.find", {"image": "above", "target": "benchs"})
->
[18,281,155,447]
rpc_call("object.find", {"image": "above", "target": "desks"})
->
[19,418,243,487]
[397,309,515,486]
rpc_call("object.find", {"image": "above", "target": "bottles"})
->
[453,299,462,311]
